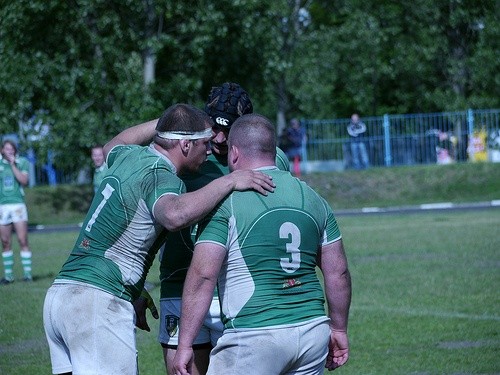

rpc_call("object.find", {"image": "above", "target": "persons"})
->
[131,82,288,375]
[277,111,500,171]
[0,140,33,285]
[168,112,353,375]
[91,145,108,194]
[42,103,276,375]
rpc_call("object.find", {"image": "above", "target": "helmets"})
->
[204,83,253,131]
